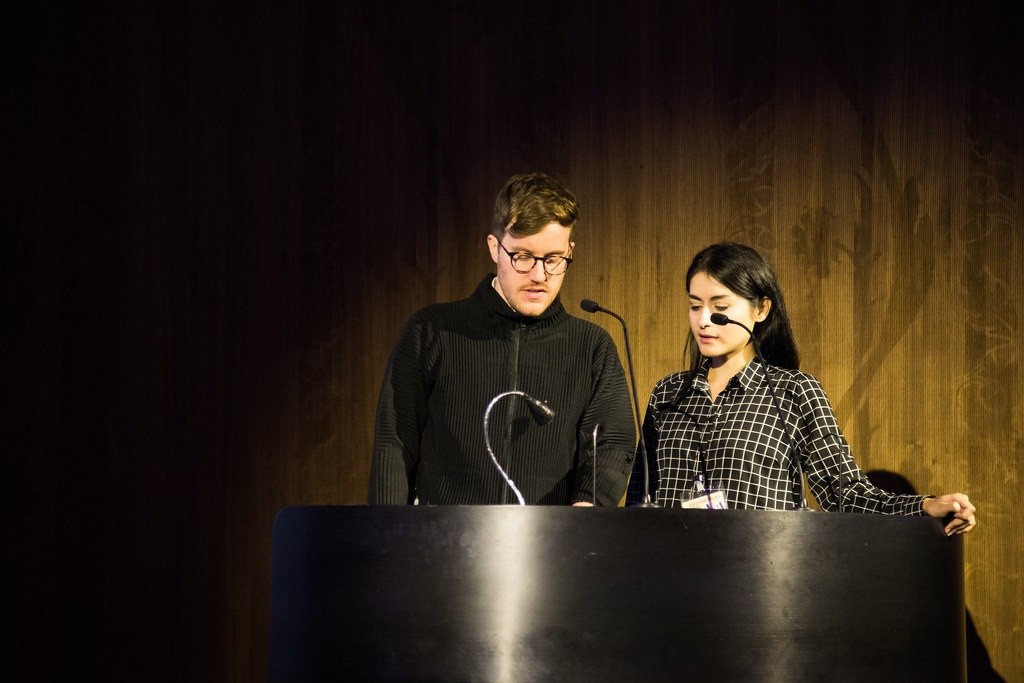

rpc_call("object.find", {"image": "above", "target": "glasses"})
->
[498,241,575,276]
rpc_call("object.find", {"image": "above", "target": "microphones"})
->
[580,299,665,507]
[710,312,822,513]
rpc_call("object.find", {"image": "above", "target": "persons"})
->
[367,173,637,506]
[624,243,976,537]
